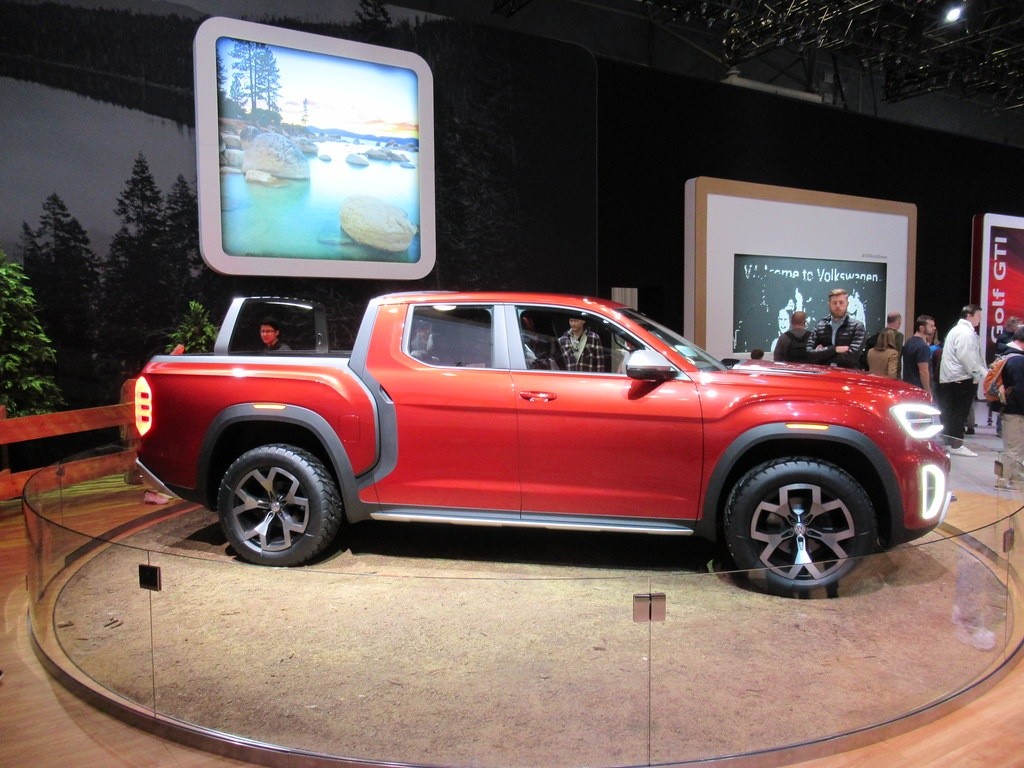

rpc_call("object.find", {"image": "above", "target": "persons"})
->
[876,311,904,379]
[260,317,292,351]
[558,318,606,373]
[135,343,185,504]
[993,326,1024,492]
[986,315,1020,437]
[867,327,900,380]
[750,348,765,361]
[774,311,814,364]
[770,308,793,353]
[939,304,983,457]
[963,331,987,435]
[805,288,866,370]
[926,331,944,401]
[902,314,936,396]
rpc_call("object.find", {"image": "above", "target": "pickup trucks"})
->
[118,290,959,597]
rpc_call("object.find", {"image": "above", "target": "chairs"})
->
[411,348,432,364]
[427,333,449,364]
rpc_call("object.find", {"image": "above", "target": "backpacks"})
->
[983,353,1023,404]
[784,331,811,363]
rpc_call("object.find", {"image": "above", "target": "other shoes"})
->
[946,445,951,451]
[993,484,1019,492]
[950,445,978,456]
[965,428,975,435]
